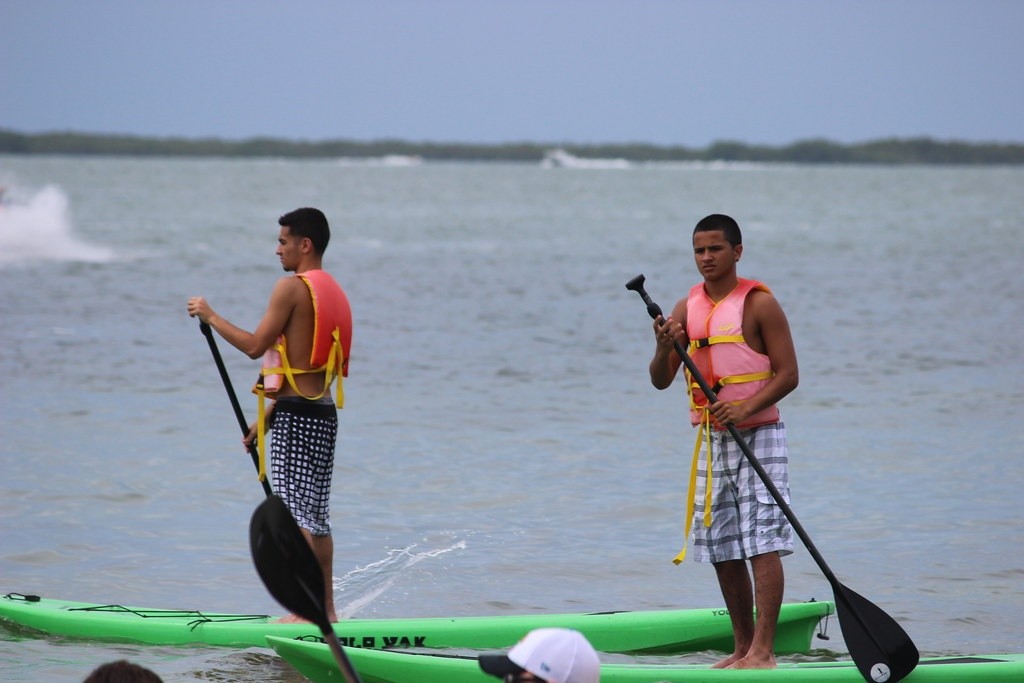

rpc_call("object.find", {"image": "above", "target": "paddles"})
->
[197,310,276,501]
[623,271,923,683]
[247,495,359,683]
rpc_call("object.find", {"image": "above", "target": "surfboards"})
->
[263,608,1023,683]
[1,581,839,654]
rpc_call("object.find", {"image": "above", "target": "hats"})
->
[479,628,600,683]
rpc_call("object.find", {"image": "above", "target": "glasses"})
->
[505,672,547,683]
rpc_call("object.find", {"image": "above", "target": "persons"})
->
[649,214,800,671]
[186,207,352,626]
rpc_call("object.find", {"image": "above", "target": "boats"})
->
[1,592,836,656]
[261,635,1024,683]
